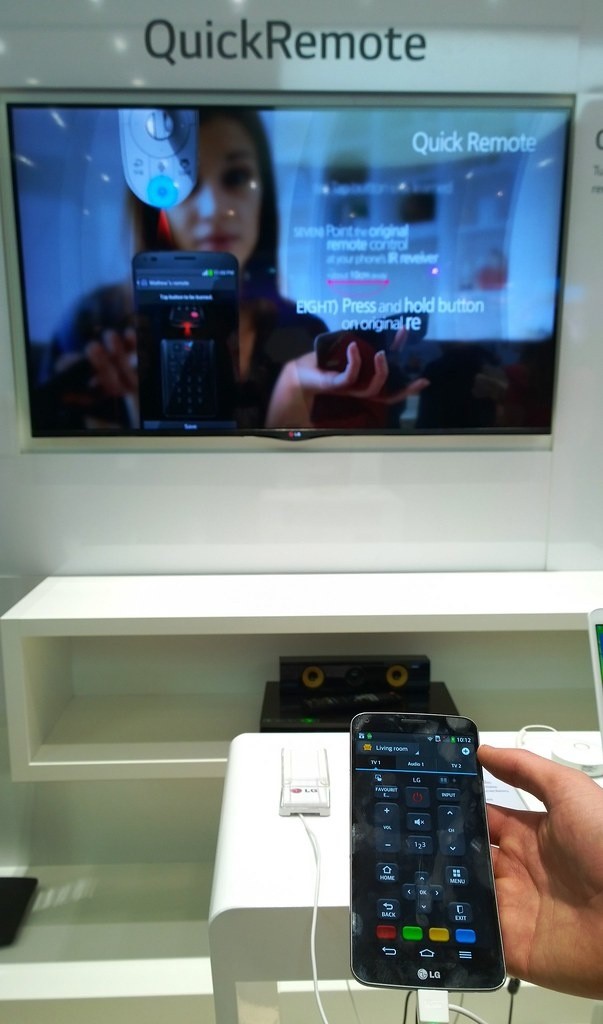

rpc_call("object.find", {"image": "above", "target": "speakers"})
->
[279,654,431,721]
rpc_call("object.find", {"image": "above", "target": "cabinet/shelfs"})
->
[0,570,603,1024]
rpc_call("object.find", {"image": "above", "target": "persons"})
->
[48,105,429,431]
[477,744,603,1001]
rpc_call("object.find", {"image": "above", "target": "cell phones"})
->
[315,310,429,369]
[131,251,240,430]
[350,712,505,992]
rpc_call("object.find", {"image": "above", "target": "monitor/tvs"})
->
[0,89,577,455]
